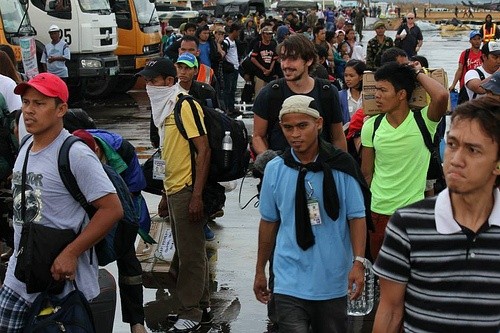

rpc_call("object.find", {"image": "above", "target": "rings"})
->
[66,275,70,279]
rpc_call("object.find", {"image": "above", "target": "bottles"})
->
[345,256,376,316]
[222,131,232,150]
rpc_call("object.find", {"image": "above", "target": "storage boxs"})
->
[132,216,176,289]
[361,68,452,116]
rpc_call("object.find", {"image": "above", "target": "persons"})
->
[164,10,365,240]
[292,6,367,41]
[369,6,382,17]
[253,48,500,332]
[0,72,124,333]
[449,14,500,103]
[454,6,474,18]
[0,45,32,189]
[424,8,427,18]
[73,129,158,333]
[367,22,393,70]
[133,58,215,333]
[396,6,400,17]
[396,13,423,56]
[413,7,416,18]
[41,25,70,81]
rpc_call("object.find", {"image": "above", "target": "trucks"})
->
[1,0,40,82]
[27,0,120,99]
[110,0,164,93]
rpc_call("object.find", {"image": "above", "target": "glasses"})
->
[276,53,302,63]
[408,17,413,20]
[143,77,165,85]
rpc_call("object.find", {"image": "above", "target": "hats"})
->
[276,26,289,34]
[176,52,198,69]
[13,73,69,103]
[470,31,481,39]
[72,129,96,152]
[373,21,385,29]
[278,95,323,136]
[215,26,225,33]
[134,57,177,79]
[481,41,500,55]
[345,20,354,26]
[261,26,273,33]
[48,25,60,33]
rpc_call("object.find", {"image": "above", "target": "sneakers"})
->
[167,318,201,333]
[168,307,214,324]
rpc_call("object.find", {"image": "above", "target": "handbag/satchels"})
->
[449,88,460,115]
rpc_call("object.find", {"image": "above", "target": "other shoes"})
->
[130,323,147,333]
[207,209,224,220]
[204,224,215,241]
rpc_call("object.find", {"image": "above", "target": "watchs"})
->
[353,256,367,269]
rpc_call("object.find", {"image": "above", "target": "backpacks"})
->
[16,134,140,267]
[173,93,251,183]
[457,68,485,105]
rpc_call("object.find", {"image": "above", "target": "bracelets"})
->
[415,69,424,75]
